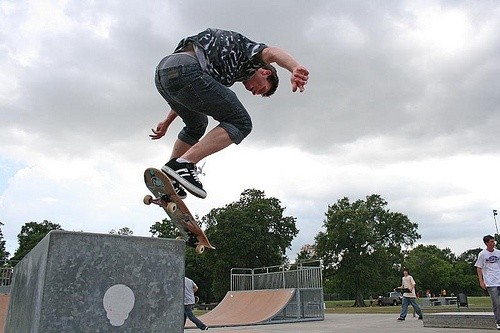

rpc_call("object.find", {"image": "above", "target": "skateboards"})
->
[192,296,199,308]
[143,168,216,254]
[394,287,411,296]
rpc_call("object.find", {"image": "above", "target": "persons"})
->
[369,295,375,307]
[441,289,447,297]
[417,290,421,299]
[149,28,309,199]
[184,277,208,330]
[397,269,423,320]
[426,288,431,298]
[378,295,383,306]
[474,234,500,330]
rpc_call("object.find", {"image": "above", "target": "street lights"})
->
[493,210,498,234]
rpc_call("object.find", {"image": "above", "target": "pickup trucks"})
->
[378,292,402,305]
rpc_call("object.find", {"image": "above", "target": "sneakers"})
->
[496,323,500,330]
[161,157,207,198]
[172,183,187,199]
